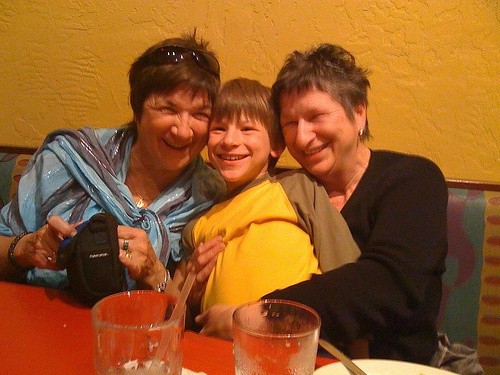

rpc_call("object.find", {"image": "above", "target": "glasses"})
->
[148,44,221,77]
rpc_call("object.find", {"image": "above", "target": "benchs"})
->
[0,146,500,375]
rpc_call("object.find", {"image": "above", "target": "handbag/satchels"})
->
[427,332,485,375]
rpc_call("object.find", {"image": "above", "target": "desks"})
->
[0,281,338,375]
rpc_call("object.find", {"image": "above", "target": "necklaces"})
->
[129,177,166,209]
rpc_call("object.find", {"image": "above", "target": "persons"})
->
[190,44,447,366]
[171,77,372,360]
[0,38,226,313]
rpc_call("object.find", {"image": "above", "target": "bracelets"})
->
[8,231,33,272]
[154,269,170,293]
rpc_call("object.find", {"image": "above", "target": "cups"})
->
[90,289,187,375]
[231,299,322,375]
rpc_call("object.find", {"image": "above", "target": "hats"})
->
[61,211,130,304]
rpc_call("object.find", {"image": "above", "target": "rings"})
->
[48,256,52,261]
[125,250,132,260]
[123,240,129,250]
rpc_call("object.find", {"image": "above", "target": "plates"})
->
[123,359,200,375]
[311,359,461,375]
